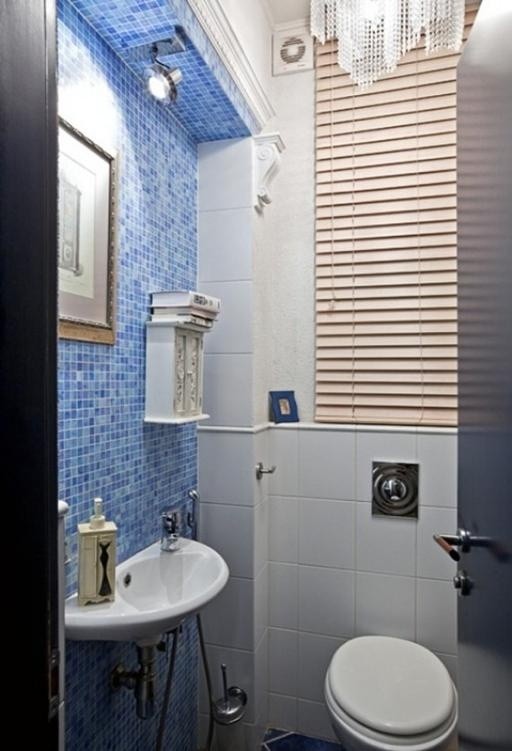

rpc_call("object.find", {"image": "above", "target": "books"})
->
[146,289,222,328]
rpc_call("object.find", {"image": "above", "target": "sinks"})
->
[65,537,230,643]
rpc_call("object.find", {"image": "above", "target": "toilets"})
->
[321,634,461,750]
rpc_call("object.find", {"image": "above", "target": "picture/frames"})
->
[54,115,120,347]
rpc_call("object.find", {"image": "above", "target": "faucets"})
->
[159,511,182,552]
